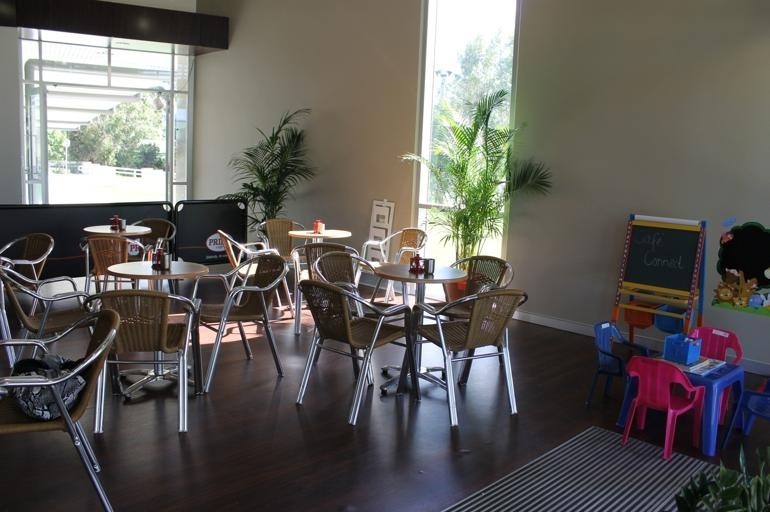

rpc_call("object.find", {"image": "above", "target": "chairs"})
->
[84,291,204,433]
[0,233,53,328]
[721,378,770,450]
[443,256,514,304]
[352,227,427,302]
[290,243,359,335]
[0,310,120,511]
[584,320,648,404]
[295,280,421,427]
[218,230,294,319]
[693,326,744,425]
[313,250,382,318]
[192,255,286,393]
[132,218,177,261]
[396,288,528,426]
[81,234,146,296]
[0,265,99,360]
[256,219,307,262]
[624,355,706,458]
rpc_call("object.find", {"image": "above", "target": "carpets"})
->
[439,427,753,512]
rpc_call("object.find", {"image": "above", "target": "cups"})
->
[425,257,435,276]
[318,223,326,234]
[163,253,172,270]
[118,220,126,230]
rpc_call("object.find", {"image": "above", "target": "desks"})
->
[288,230,352,280]
[84,225,151,236]
[618,353,746,455]
[376,264,468,396]
[106,261,209,400]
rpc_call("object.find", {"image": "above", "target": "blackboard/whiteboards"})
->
[620,219,702,295]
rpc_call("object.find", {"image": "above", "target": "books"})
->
[655,355,727,375]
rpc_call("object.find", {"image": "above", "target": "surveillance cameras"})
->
[153,92,164,110]
